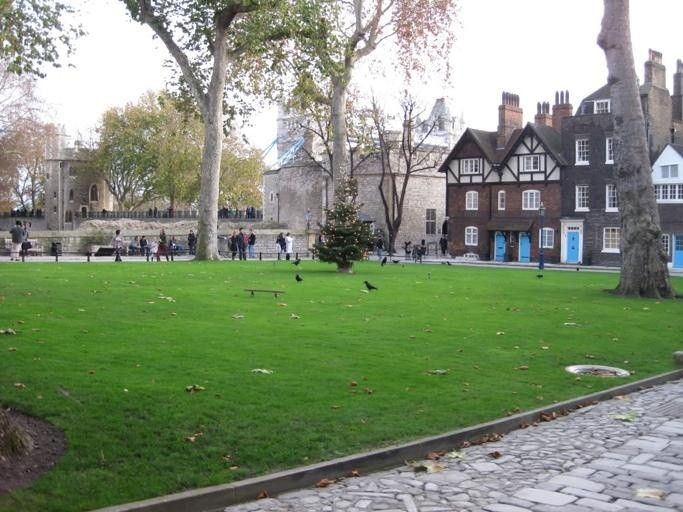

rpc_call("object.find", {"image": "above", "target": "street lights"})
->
[539,203,546,271]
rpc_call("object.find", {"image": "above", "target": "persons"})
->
[7,206,296,263]
[316,230,446,260]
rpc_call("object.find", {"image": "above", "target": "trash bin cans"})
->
[52,242,62,256]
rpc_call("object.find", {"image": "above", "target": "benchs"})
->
[123,240,158,255]
[244,289,288,299]
[4,238,45,257]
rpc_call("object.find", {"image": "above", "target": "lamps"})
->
[491,160,502,171]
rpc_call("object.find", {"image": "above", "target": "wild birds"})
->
[296,274,303,283]
[292,259,301,266]
[536,275,543,278]
[392,260,400,264]
[364,281,378,292]
[381,257,387,267]
[447,261,452,266]
